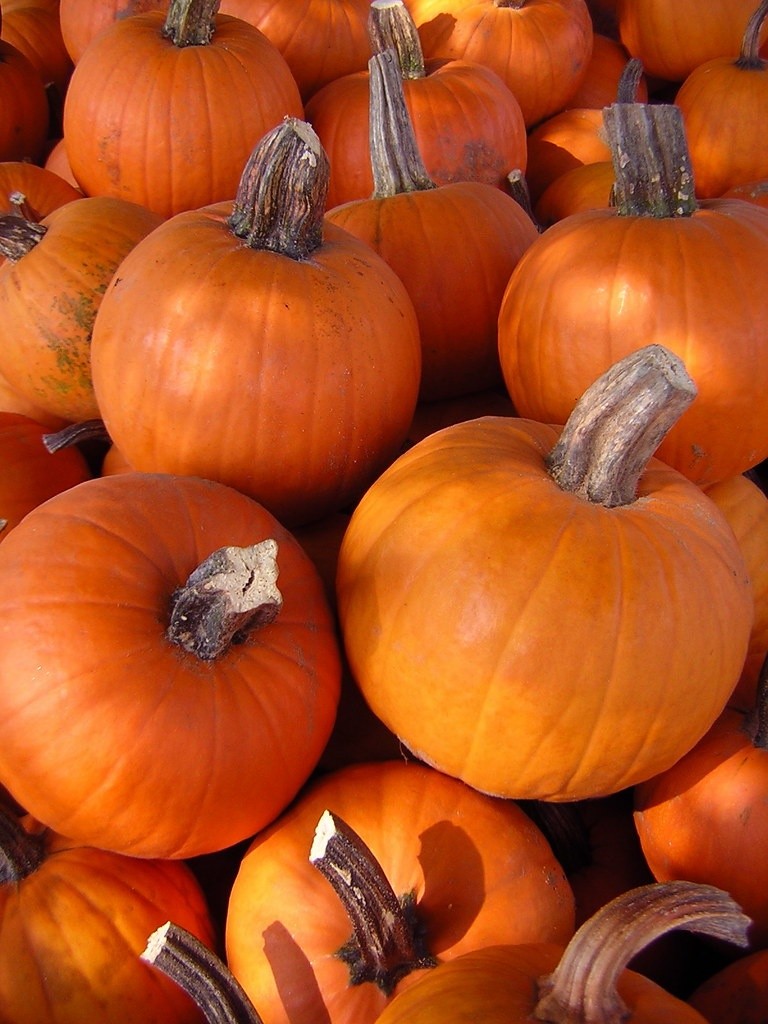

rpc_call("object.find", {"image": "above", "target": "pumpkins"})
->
[0,2,768,1024]
